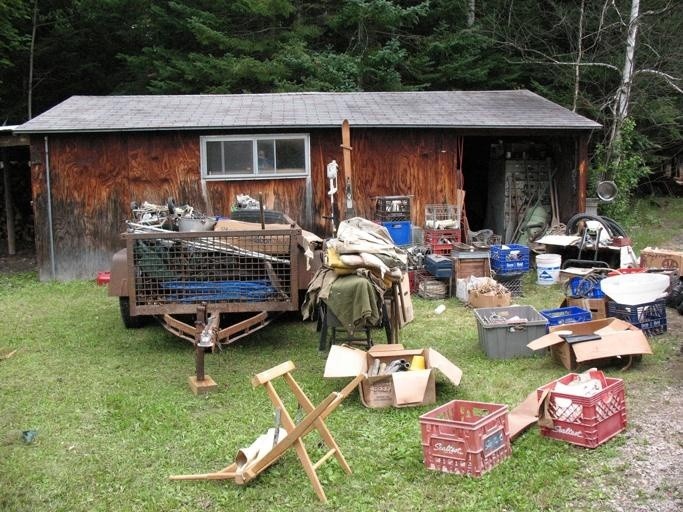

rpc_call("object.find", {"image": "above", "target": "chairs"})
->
[170,360,365,503]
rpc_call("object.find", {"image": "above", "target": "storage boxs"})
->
[325,346,463,408]
[641,250,683,276]
[560,267,593,286]
[382,222,410,245]
[427,254,452,277]
[455,277,495,303]
[529,318,653,371]
[476,306,546,360]
[567,297,608,318]
[470,287,510,307]
[213,220,289,256]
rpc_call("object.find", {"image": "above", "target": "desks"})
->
[451,250,494,294]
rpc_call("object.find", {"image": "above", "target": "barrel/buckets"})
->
[534,253,562,286]
[177,217,206,233]
